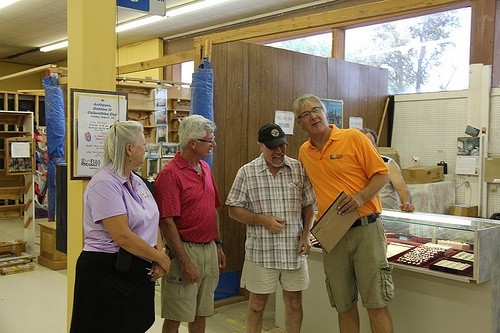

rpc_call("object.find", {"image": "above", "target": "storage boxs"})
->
[449,204,478,216]
[402,165,444,184]
[485,157,500,182]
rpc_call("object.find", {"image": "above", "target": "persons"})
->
[225,123,315,333]
[154,115,226,333]
[70,121,171,333]
[360,128,415,212]
[293,94,393,333]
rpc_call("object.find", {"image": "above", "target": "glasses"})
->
[188,135,216,144]
[297,106,321,119]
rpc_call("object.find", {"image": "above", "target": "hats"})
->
[258,123,288,149]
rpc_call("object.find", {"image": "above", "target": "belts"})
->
[351,214,379,228]
[182,239,210,244]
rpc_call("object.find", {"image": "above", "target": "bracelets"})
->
[215,240,223,245]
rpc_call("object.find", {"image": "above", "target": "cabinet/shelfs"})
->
[117,78,193,181]
[276,208,500,333]
[0,111,67,271]
